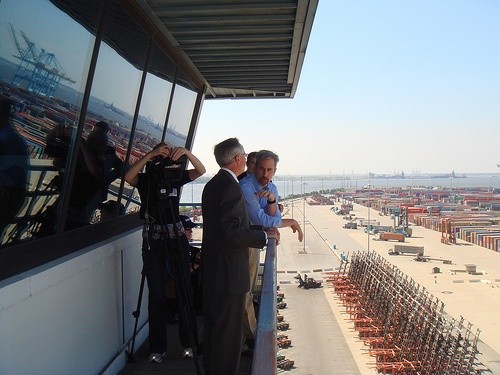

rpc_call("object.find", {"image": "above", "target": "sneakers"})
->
[149,352,167,364]
[183,347,194,360]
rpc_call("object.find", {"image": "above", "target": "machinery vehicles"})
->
[294,273,322,289]
[392,206,412,238]
[341,196,353,210]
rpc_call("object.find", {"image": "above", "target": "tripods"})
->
[128,199,202,375]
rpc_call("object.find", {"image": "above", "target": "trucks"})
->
[350,217,381,226]
[343,214,355,219]
[364,225,392,234]
[373,232,404,242]
[358,219,380,226]
[305,195,334,205]
[388,245,424,256]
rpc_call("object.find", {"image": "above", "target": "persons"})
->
[124,142,206,364]
[239,150,303,360]
[235,151,260,182]
[0,91,127,239]
[201,136,280,375]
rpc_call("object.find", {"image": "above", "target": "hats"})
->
[179,215,197,228]
